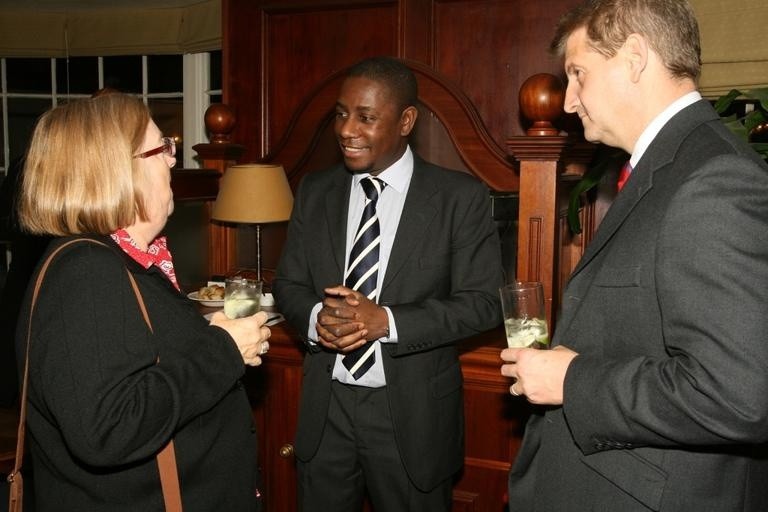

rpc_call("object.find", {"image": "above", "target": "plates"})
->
[186,291,224,307]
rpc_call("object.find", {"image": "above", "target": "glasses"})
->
[131,136,176,158]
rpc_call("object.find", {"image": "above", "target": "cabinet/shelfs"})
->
[193,302,523,512]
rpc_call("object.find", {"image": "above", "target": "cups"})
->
[221,276,262,318]
[498,280,549,349]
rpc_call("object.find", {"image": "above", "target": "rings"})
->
[510,385,518,396]
[261,344,267,354]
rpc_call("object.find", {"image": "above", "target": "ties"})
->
[342,177,388,380]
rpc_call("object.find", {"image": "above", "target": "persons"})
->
[15,88,271,512]
[275,59,511,512]
[501,0,768,512]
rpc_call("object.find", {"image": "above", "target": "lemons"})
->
[536,318,552,345]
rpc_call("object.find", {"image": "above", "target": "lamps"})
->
[205,161,298,308]
